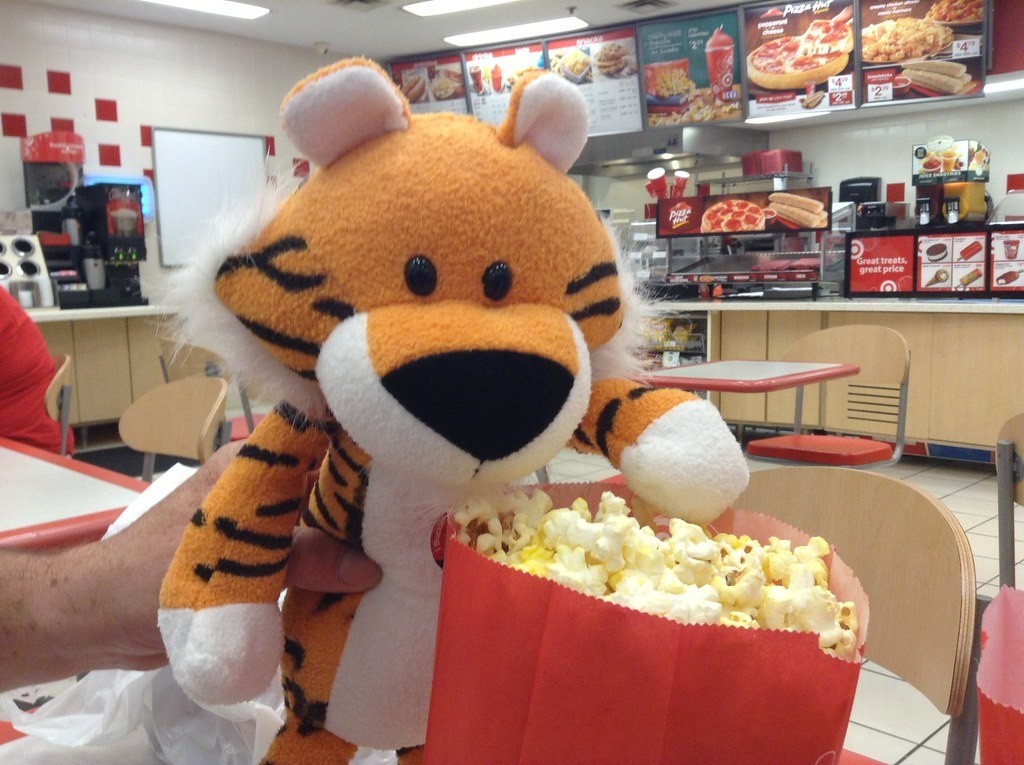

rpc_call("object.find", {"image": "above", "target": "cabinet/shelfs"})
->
[127,315,240,403]
[36,320,132,429]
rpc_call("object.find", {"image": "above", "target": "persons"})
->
[0,286,74,459]
[0,436,383,694]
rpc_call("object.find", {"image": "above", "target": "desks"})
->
[638,356,861,440]
[0,433,151,556]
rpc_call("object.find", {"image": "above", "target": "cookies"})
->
[593,43,625,73]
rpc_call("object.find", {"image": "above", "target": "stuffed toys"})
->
[155,57,750,765]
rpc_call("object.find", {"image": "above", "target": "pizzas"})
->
[747,19,852,89]
[701,201,765,232]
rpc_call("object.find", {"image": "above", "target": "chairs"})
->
[44,350,73,458]
[155,333,255,434]
[747,324,913,473]
[976,411,1024,765]
[741,458,993,765]
[118,375,227,481]
